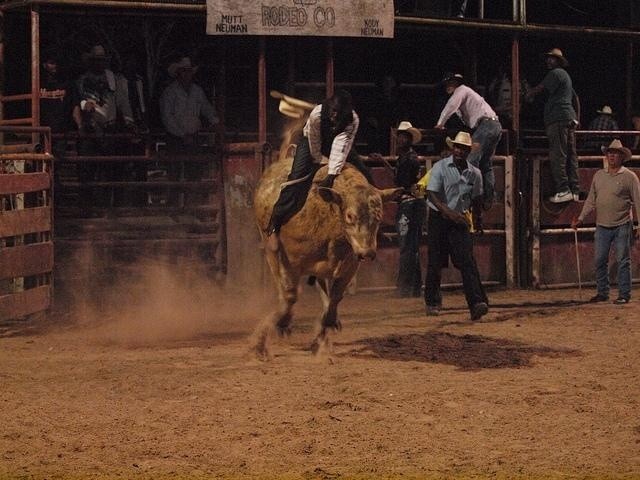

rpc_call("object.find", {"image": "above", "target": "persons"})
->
[525,48,580,204]
[427,131,490,320]
[434,73,502,214]
[572,139,640,303]
[626,104,640,150]
[413,138,475,238]
[481,59,533,123]
[264,91,376,237]
[585,106,619,150]
[371,120,428,299]
[40,43,222,214]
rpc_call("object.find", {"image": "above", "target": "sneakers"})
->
[426,305,441,315]
[549,191,579,203]
[469,303,488,320]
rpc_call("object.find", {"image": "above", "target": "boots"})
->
[267,217,281,253]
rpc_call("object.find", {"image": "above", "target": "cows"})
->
[252,111,407,330]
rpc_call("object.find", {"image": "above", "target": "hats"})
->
[80,44,112,63]
[545,48,568,62]
[446,132,480,149]
[597,106,617,116]
[440,70,461,88]
[167,56,198,79]
[390,121,422,145]
[601,139,632,162]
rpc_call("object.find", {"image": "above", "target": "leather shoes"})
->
[614,298,627,304]
